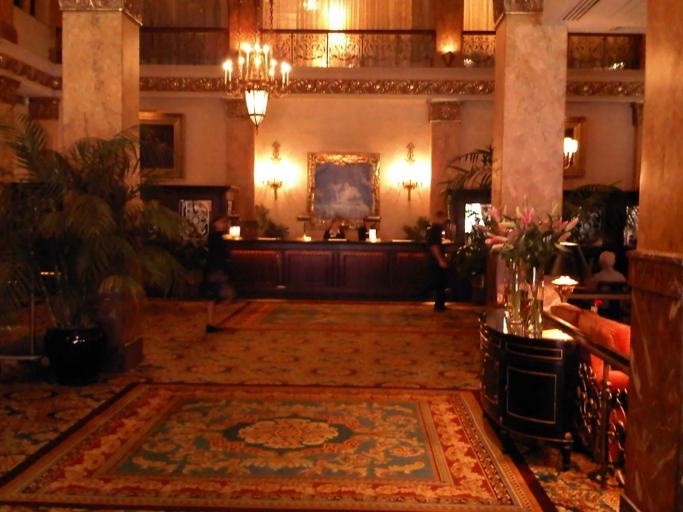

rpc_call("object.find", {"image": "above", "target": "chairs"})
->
[594,280,631,295]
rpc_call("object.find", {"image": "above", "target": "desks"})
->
[473,309,580,474]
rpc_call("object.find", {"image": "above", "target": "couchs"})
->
[545,298,632,483]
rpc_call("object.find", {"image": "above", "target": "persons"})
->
[357,215,377,241]
[424,210,450,311]
[337,178,362,204]
[322,218,344,239]
[202,215,237,333]
[321,178,343,203]
[584,249,626,288]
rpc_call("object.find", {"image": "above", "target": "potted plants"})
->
[0,94,233,381]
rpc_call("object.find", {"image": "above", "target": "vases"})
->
[502,251,547,339]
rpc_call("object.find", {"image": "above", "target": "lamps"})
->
[218,36,294,128]
[400,142,419,202]
[267,140,284,198]
[562,137,579,168]
[550,274,579,303]
[441,50,455,66]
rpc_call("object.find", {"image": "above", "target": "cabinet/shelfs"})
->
[276,242,389,303]
[385,242,431,303]
[226,237,281,299]
[6,180,237,304]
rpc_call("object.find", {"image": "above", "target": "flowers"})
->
[468,200,585,266]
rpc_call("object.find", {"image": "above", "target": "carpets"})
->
[0,378,559,510]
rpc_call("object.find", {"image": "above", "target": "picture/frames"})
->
[138,109,187,179]
[306,151,380,231]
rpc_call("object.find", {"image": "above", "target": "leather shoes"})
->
[206,325,224,332]
